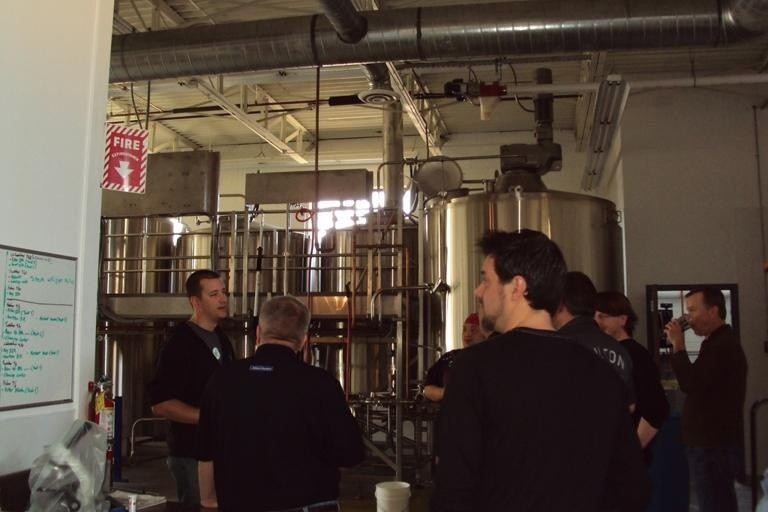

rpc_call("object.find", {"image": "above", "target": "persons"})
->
[426,226,653,511]
[423,310,487,402]
[553,271,639,414]
[664,286,749,510]
[147,270,236,506]
[596,290,671,470]
[195,294,366,512]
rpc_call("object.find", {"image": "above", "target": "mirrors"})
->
[645,283,739,380]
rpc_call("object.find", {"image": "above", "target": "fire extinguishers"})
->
[88,375,115,463]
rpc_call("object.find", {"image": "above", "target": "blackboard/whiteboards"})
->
[0,243,78,412]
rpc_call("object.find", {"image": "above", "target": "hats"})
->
[464,312,479,324]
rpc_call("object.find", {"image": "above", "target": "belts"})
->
[283,501,338,512]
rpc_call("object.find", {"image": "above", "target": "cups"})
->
[372,480,411,511]
[677,314,693,332]
